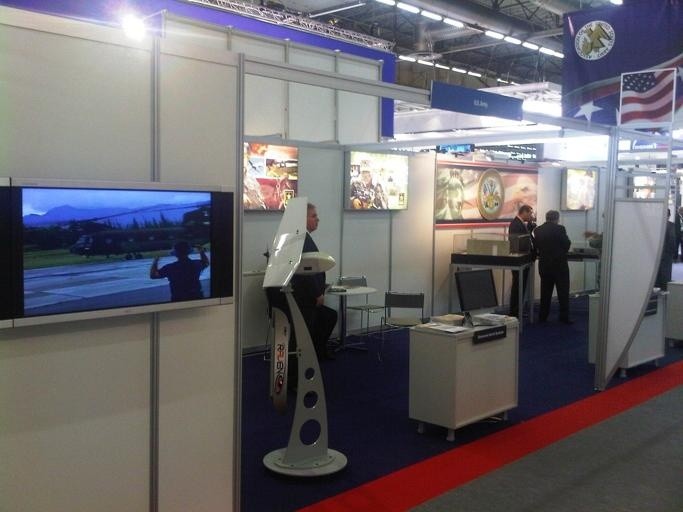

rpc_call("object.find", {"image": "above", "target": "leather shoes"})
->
[560,320,573,326]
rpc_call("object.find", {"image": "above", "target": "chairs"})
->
[340,275,424,361]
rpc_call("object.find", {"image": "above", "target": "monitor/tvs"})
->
[0,177,236,331]
[454,268,499,329]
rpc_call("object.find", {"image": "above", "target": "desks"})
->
[324,286,378,352]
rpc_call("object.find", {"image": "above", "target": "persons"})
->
[350,171,389,210]
[673,207,683,262]
[268,204,338,396]
[589,232,603,248]
[150,242,209,301]
[663,209,677,282]
[509,206,537,317]
[534,210,574,326]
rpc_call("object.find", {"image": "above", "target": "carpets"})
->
[242,291,683,511]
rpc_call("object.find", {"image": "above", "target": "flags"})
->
[562,0,683,128]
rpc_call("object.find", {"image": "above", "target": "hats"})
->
[170,242,194,257]
